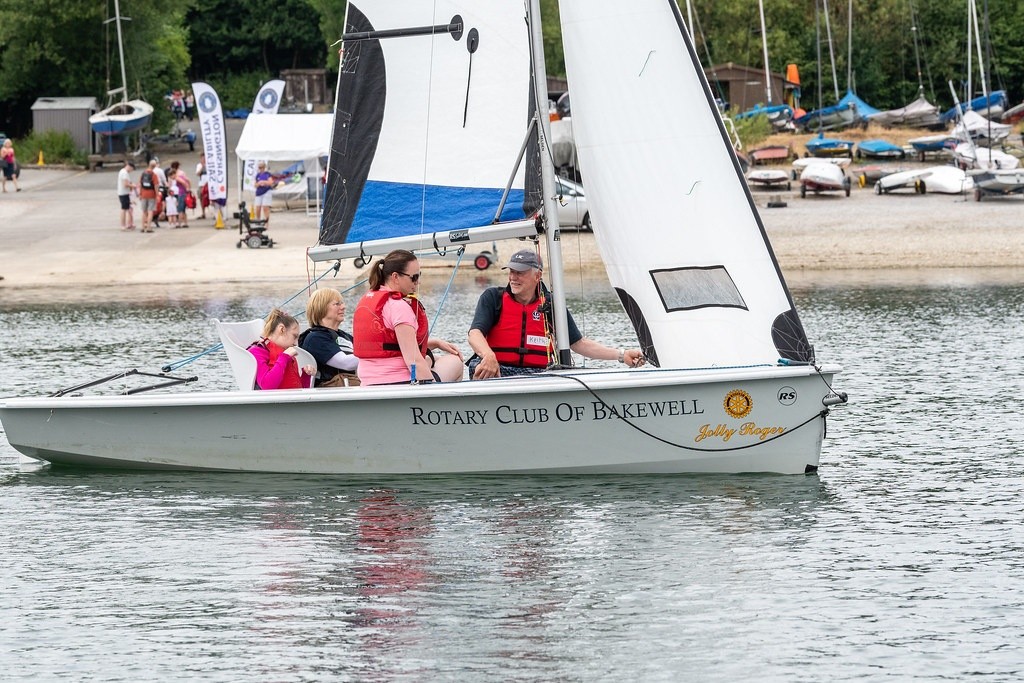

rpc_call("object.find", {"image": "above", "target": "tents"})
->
[234,112,334,229]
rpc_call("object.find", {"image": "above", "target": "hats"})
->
[501,248,543,271]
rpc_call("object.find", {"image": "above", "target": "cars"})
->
[555,176,593,231]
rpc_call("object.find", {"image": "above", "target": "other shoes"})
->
[117,213,215,234]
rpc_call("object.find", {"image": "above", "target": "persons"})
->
[118,161,138,232]
[352,250,464,386]
[179,133,196,152]
[299,287,358,388]
[322,166,327,187]
[139,153,216,233]
[463,248,646,382]
[1,139,22,192]
[255,162,273,228]
[165,89,196,122]
[246,306,318,390]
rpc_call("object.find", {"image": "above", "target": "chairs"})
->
[210,318,317,390]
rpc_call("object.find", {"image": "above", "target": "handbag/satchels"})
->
[186,190,196,209]
[0,156,8,169]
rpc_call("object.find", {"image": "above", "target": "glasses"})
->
[390,270,421,282]
[327,299,345,307]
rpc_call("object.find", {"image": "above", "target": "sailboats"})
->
[746,0,1024,201]
[88,0,154,136]
[0,0,848,476]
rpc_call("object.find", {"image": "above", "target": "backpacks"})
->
[200,183,208,207]
[141,172,155,189]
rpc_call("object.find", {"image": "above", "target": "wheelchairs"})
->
[233,201,276,248]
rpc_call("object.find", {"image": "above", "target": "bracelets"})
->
[618,348,625,362]
